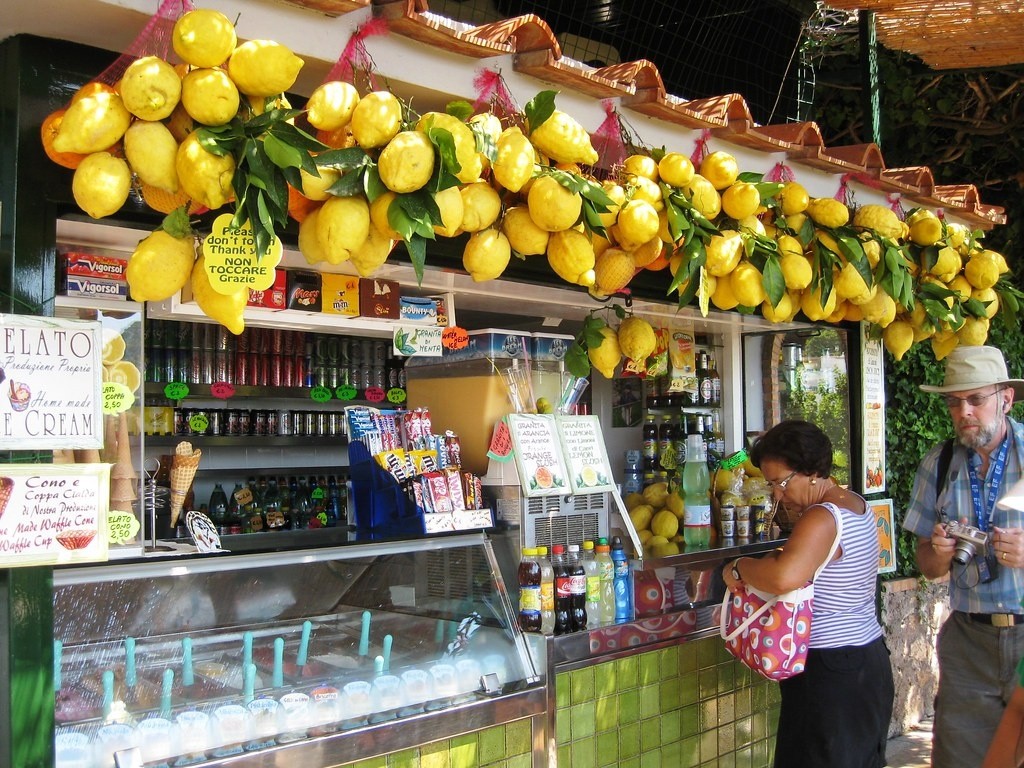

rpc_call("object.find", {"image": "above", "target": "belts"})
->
[964,613,1024,626]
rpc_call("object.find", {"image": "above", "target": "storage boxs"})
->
[286,271,321,313]
[57,253,128,279]
[359,277,400,318]
[322,273,361,316]
[244,268,287,308]
[60,275,128,302]
[399,296,439,326]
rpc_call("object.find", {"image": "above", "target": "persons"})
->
[721,421,896,768]
[900,346,1024,767]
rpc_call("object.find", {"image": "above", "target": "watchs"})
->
[731,556,744,581]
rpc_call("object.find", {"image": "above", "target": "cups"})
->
[720,505,765,538]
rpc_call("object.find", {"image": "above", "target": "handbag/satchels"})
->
[720,502,842,681]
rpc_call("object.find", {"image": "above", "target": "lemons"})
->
[626,457,769,547]
[52,8,1009,379]
[527,398,553,414]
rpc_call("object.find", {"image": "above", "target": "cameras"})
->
[944,520,989,566]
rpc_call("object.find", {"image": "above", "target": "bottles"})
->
[198,472,351,536]
[518,536,635,637]
[622,350,724,495]
[682,434,712,553]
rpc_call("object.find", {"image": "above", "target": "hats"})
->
[919,346,1024,402]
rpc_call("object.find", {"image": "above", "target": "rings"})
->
[733,587,738,592]
[932,544,937,551]
[1002,553,1006,561]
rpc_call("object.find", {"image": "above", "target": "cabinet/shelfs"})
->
[144,319,407,447]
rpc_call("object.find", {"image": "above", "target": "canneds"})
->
[145,318,406,436]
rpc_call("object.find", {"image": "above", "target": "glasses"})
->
[767,471,798,492]
[942,386,1011,408]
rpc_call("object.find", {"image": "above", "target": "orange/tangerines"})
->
[40,64,363,223]
